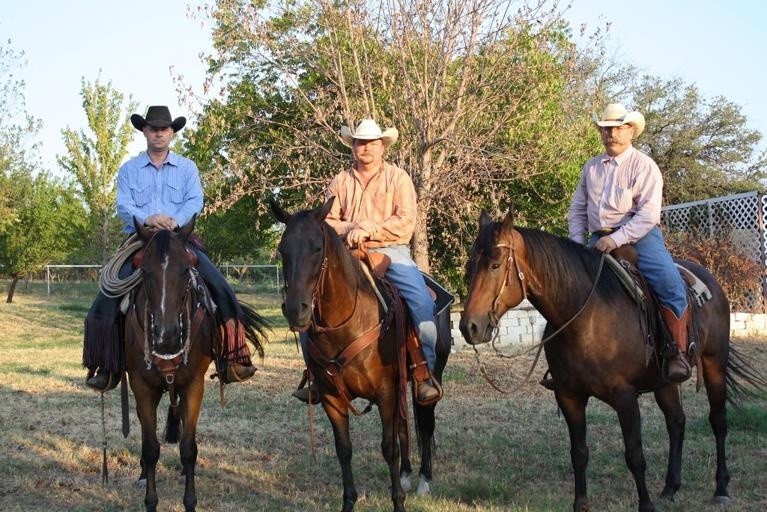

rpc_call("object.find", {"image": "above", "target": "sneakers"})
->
[415,379,439,403]
[223,360,257,384]
[291,382,321,405]
[84,367,121,390]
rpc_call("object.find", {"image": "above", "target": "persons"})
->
[85,104,256,390]
[539,102,691,388]
[288,118,440,407]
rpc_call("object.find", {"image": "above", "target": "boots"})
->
[538,378,554,391]
[659,302,689,380]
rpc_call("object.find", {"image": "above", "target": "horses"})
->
[267,194,453,512]
[122,211,276,512]
[459,201,767,511]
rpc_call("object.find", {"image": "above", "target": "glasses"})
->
[599,125,632,132]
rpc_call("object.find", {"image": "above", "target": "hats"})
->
[130,105,186,134]
[339,119,399,151]
[591,103,645,141]
